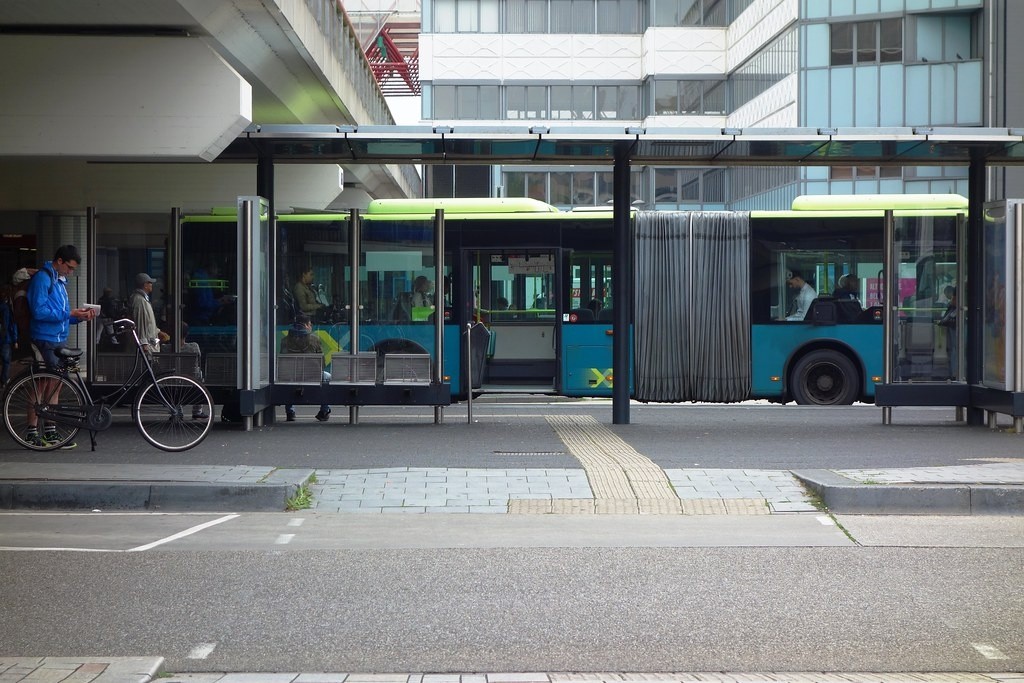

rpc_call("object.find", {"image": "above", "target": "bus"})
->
[166,190,990,405]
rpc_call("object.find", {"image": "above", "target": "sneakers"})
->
[42,432,77,450]
[18,433,52,450]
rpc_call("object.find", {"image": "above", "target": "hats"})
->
[133,272,157,290]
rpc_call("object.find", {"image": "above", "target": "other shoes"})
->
[316,409,330,421]
[168,413,183,421]
[286,413,295,421]
[192,413,209,420]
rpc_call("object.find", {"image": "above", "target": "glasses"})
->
[64,261,77,271]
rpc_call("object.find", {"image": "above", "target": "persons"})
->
[588,299,603,317]
[833,274,873,316]
[0,295,19,389]
[26,245,96,450]
[95,286,120,346]
[280,316,332,423]
[774,271,819,321]
[411,276,436,323]
[493,297,513,320]
[162,320,210,422]
[126,274,169,356]
[292,267,326,325]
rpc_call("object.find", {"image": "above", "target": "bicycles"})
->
[0,318,214,453]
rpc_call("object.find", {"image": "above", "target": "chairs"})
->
[599,307,616,322]
[282,283,330,326]
[571,309,594,322]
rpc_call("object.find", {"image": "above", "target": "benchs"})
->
[92,352,451,432]
